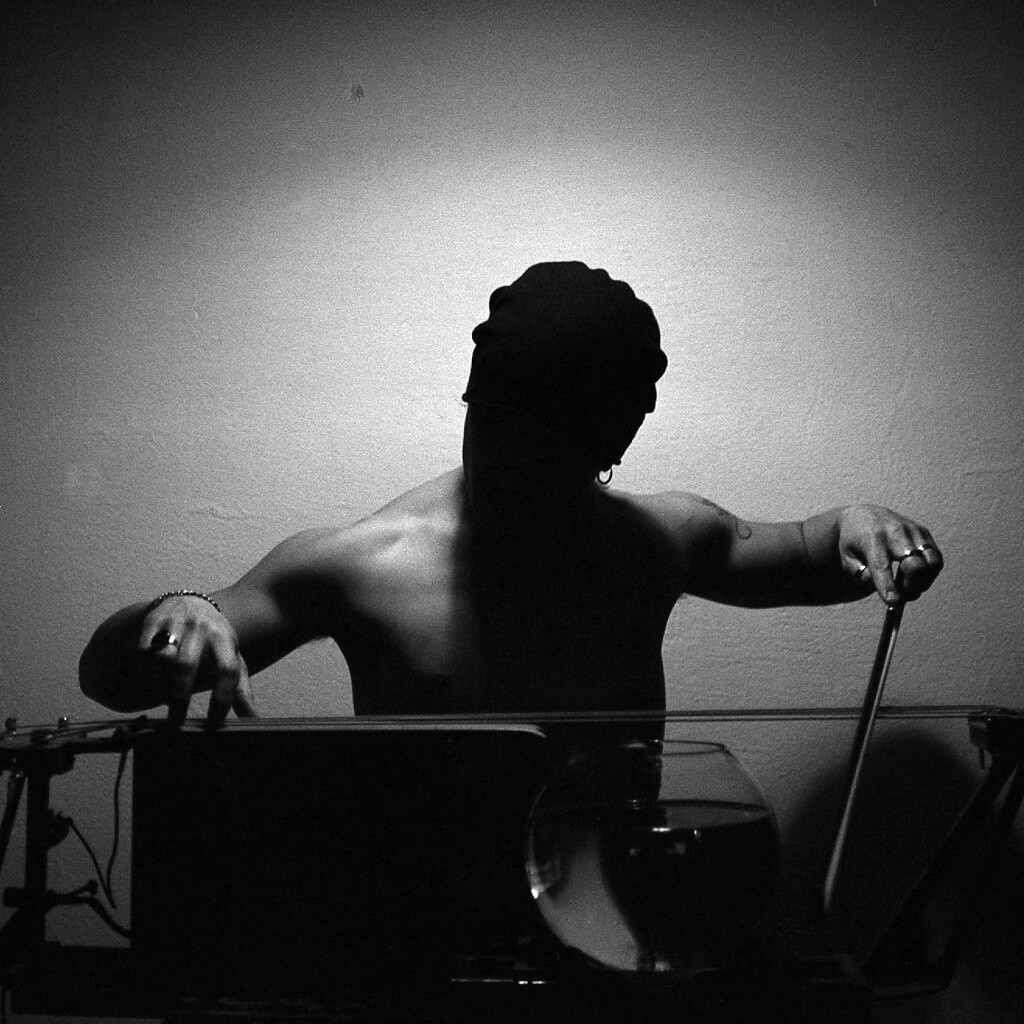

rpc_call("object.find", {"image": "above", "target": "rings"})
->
[856,564,868,579]
[898,542,935,566]
[151,630,181,654]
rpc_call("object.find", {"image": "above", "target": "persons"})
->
[79,259,945,800]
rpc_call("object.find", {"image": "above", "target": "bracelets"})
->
[145,589,221,616]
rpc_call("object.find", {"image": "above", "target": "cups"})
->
[525,739,782,997]
